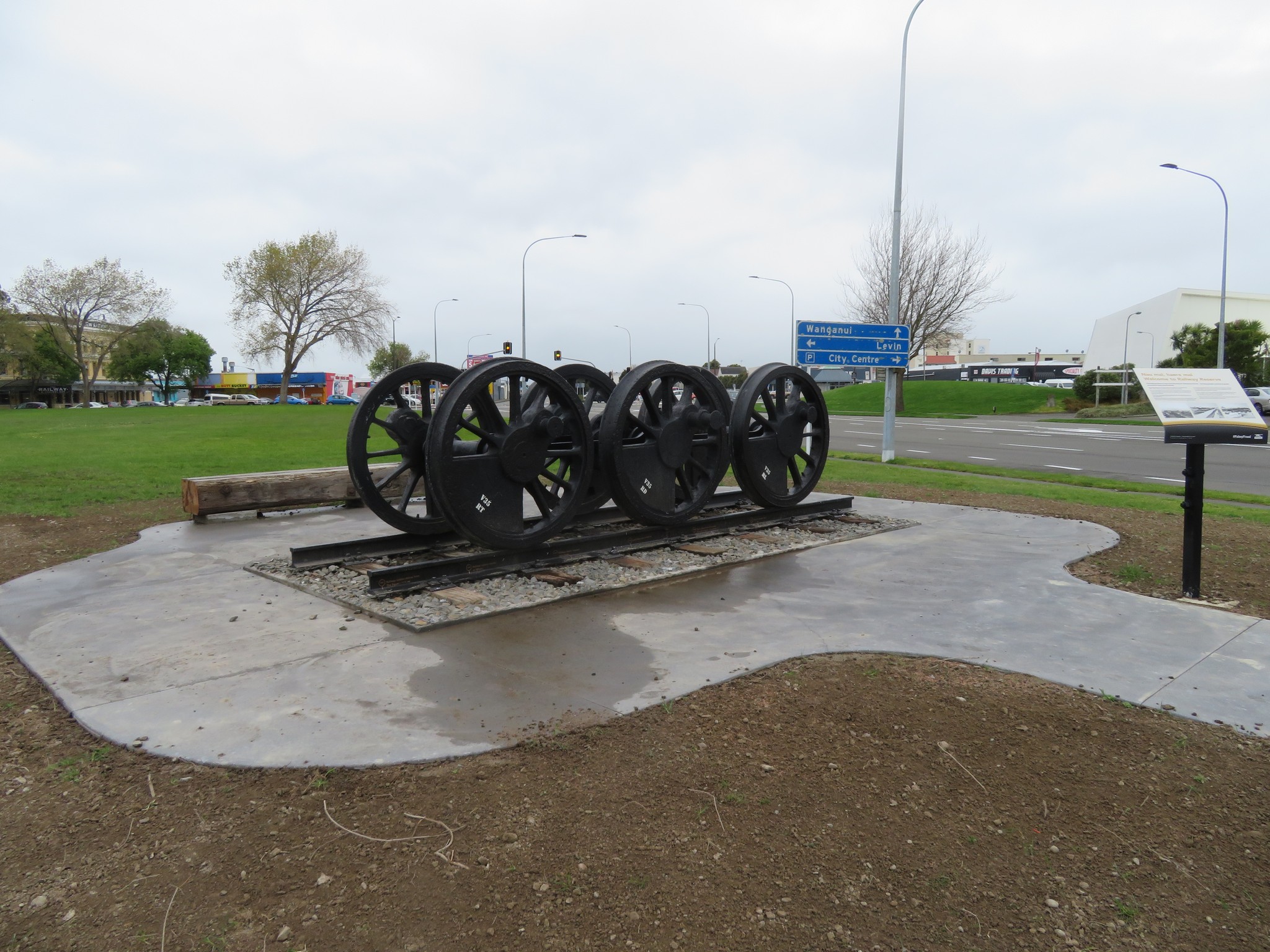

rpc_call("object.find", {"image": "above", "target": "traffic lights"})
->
[556,351,560,360]
[505,342,511,354]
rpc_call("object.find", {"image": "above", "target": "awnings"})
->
[0,380,75,404]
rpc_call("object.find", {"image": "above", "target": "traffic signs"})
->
[796,320,910,368]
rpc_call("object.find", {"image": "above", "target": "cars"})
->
[583,391,604,403]
[770,389,791,399]
[672,390,697,400]
[69,394,361,409]
[1242,387,1270,414]
[381,391,445,406]
[13,402,48,409]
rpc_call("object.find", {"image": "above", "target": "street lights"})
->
[434,299,459,406]
[678,303,710,371]
[1121,311,1142,404]
[614,326,631,371]
[393,317,401,370]
[749,276,793,366]
[1137,331,1154,368]
[714,338,720,375]
[1159,164,1228,369]
[523,235,588,395]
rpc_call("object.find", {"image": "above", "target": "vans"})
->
[1005,379,1074,389]
